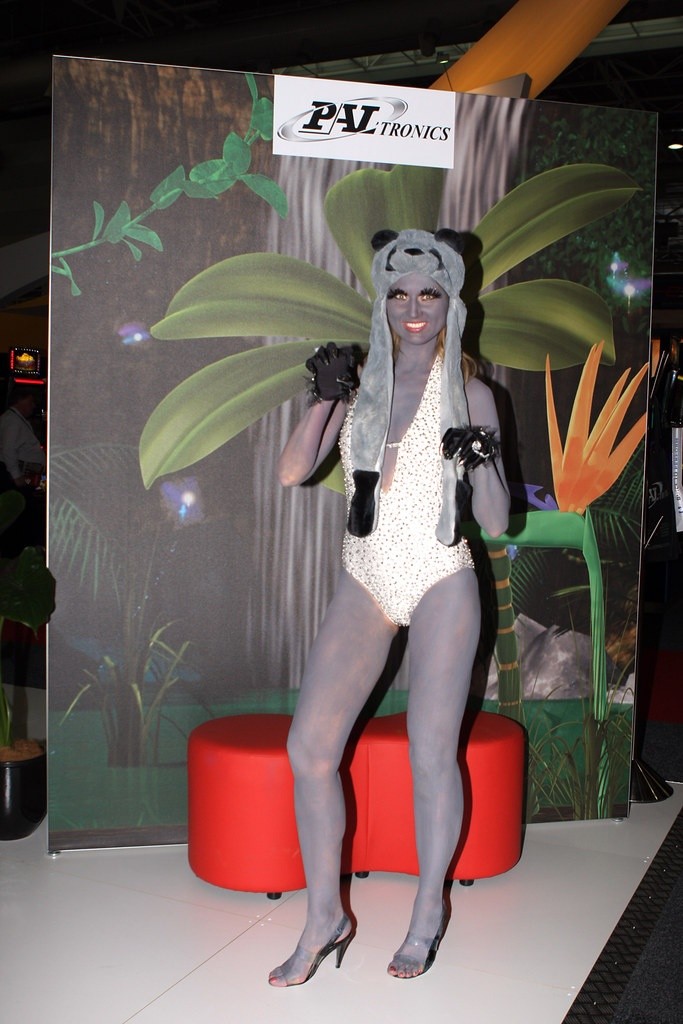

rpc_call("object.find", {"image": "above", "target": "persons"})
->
[0,385,46,499]
[267,225,511,988]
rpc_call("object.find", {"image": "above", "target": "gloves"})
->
[443,428,497,470]
[306,342,360,400]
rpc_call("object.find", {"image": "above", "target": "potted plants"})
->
[1,494,57,841]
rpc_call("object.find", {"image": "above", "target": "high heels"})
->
[272,913,356,987]
[390,897,451,978]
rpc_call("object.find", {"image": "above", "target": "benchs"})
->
[187,706,527,898]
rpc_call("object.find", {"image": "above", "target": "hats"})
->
[349,229,469,546]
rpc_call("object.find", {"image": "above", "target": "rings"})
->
[472,441,490,458]
